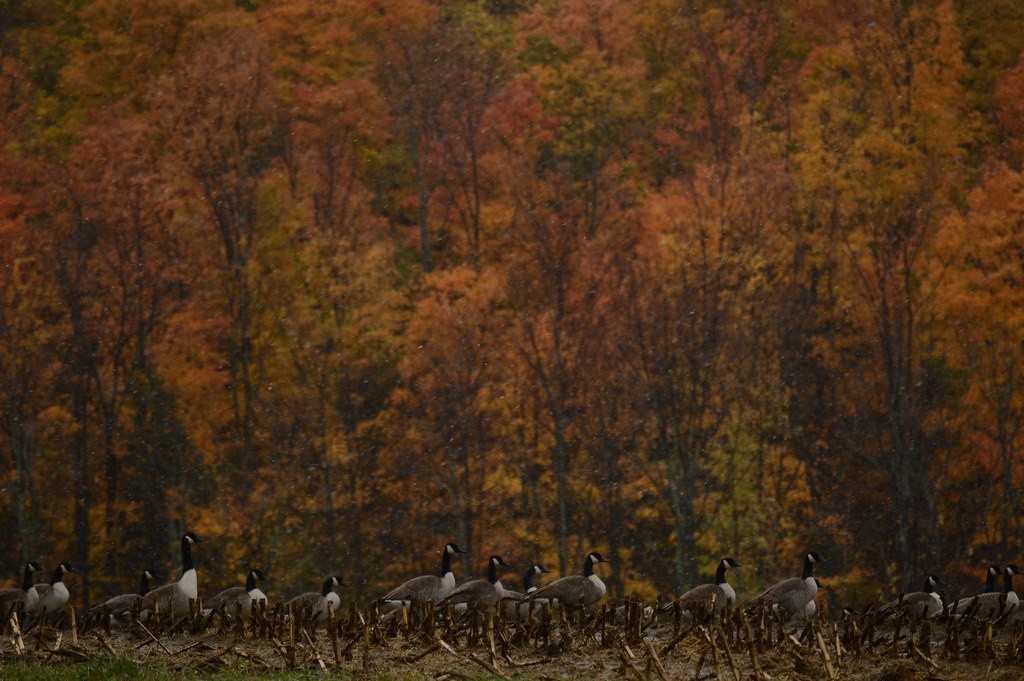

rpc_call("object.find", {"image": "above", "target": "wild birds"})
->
[0,526,1023,681]
